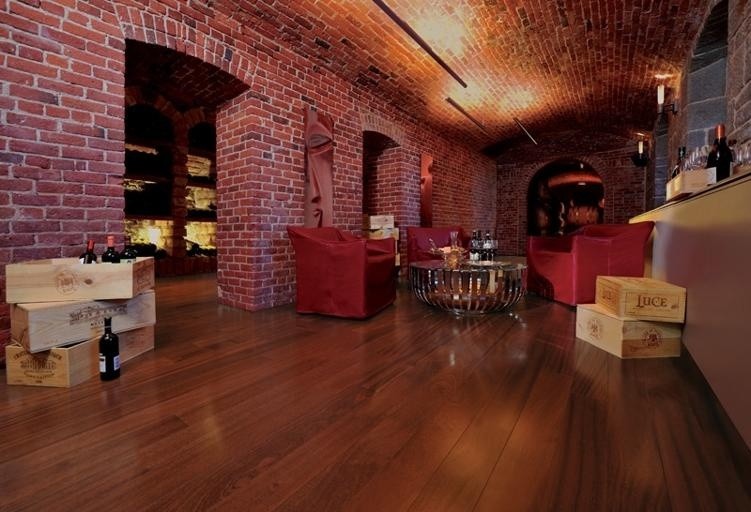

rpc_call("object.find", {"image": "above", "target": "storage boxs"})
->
[594,275,689,329]
[9,287,158,355]
[5,255,157,306]
[361,214,402,269]
[664,170,710,202]
[5,324,156,390]
[573,301,684,363]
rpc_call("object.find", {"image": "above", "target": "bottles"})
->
[99,316,121,381]
[103,236,121,263]
[442,231,467,270]
[79,240,97,264]
[120,236,138,259]
[469,229,499,262]
[707,124,734,182]
[672,146,687,179]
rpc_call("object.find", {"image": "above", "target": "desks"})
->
[407,260,529,318]
[469,245,496,260]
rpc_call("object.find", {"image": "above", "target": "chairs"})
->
[523,221,655,310]
[284,224,398,322]
[405,225,475,281]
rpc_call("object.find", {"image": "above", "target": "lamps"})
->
[637,138,655,159]
[655,82,680,116]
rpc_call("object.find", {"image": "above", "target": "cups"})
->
[688,145,707,171]
